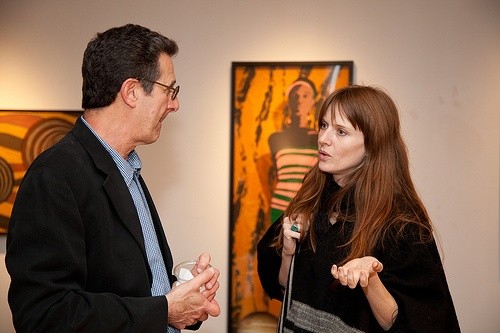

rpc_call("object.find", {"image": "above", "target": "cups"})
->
[173,259,209,293]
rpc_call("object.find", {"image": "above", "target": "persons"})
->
[258,84,461,333]
[5,23,221,333]
[267,77,320,317]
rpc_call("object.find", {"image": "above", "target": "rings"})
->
[345,274,348,276]
[291,223,298,231]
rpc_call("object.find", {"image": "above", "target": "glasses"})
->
[136,77,180,100]
